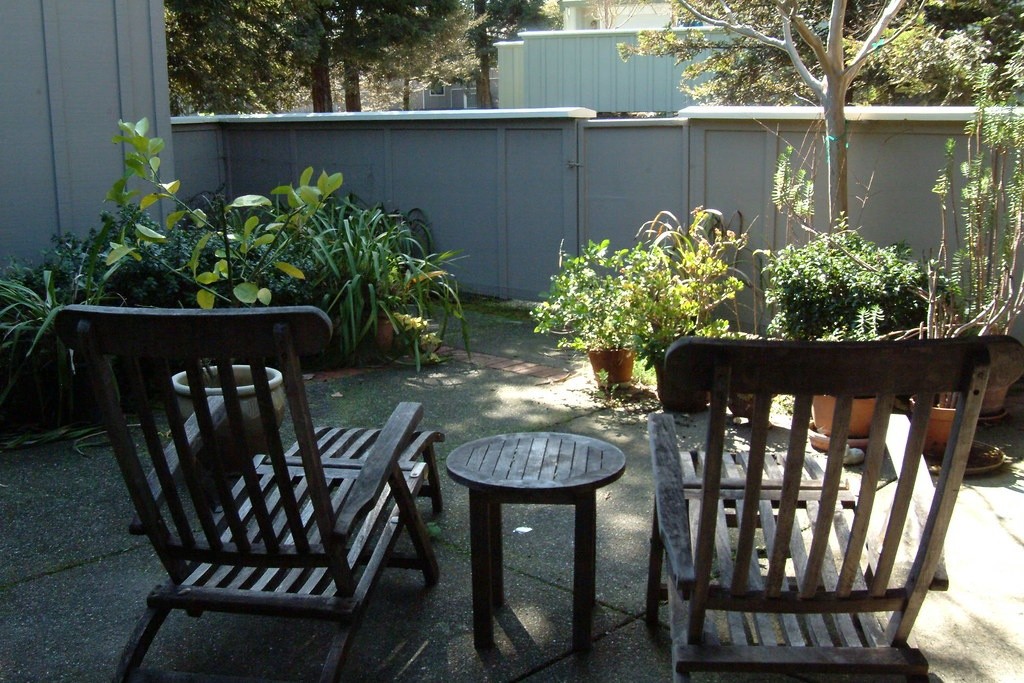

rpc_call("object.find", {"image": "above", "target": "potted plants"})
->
[0,114,475,463]
[530,59,1024,478]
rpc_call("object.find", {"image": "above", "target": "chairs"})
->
[54,304,445,683]
[642,335,992,683]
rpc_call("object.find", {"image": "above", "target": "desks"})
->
[444,430,626,658]
